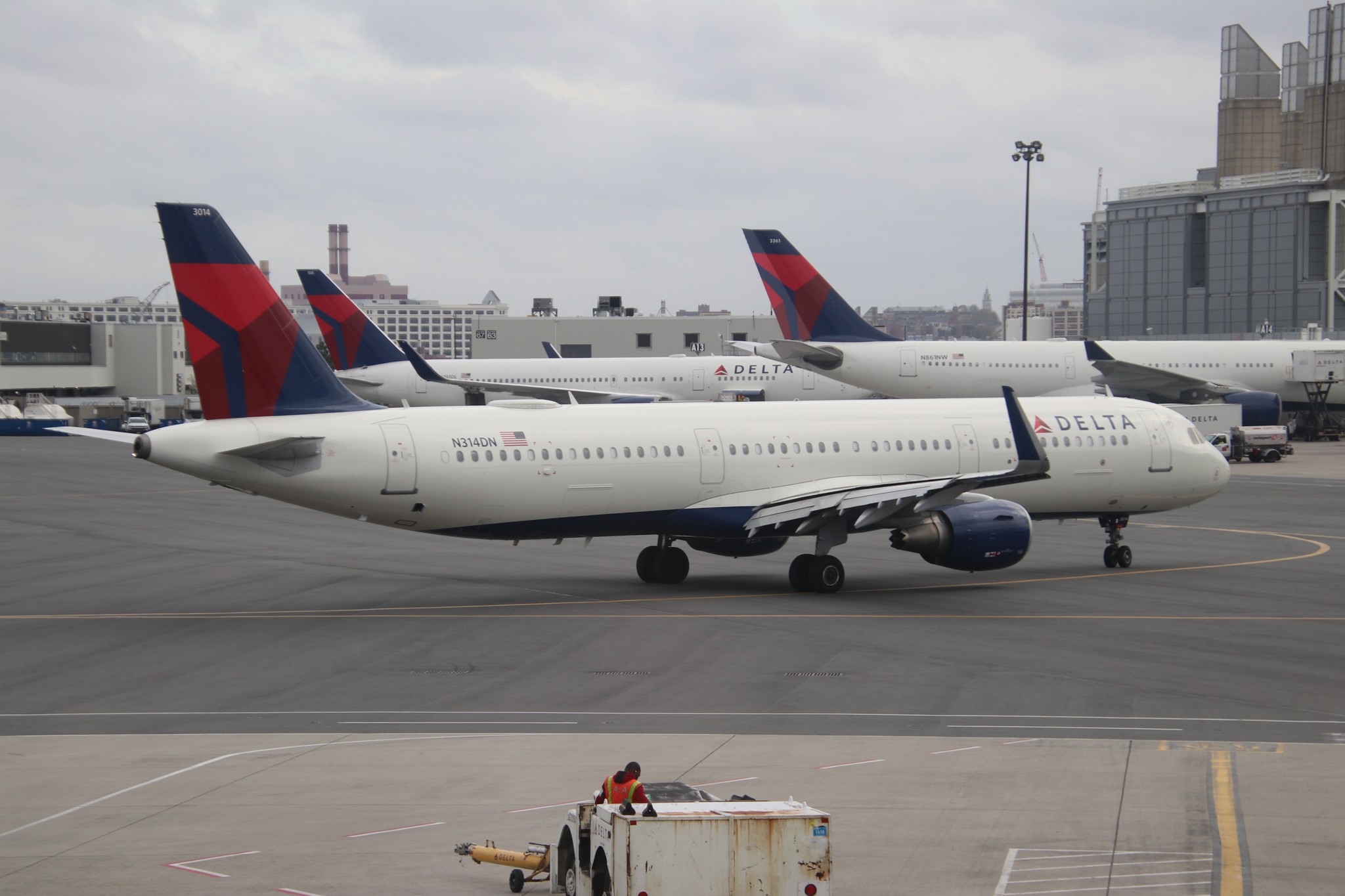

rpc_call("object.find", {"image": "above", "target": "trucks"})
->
[1203,424,1294,463]
[119,396,166,430]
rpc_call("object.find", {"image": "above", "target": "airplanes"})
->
[39,202,1234,595]
[295,228,1345,445]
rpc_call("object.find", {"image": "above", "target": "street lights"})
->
[1011,140,1045,341]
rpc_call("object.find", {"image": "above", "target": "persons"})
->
[594,761,650,804]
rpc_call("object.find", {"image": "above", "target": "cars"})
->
[121,416,151,434]
